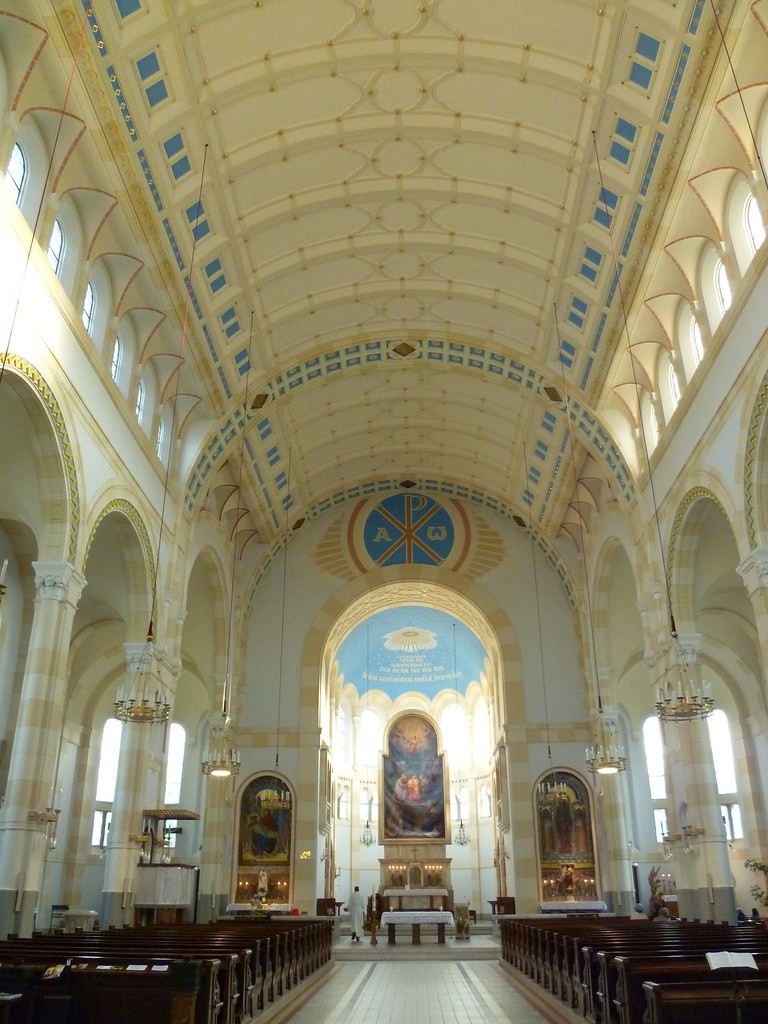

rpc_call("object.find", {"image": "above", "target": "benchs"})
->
[0,918,334,1024]
[499,917,768,1024]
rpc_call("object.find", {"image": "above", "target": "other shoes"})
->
[352,933,356,939]
[357,937,359,941]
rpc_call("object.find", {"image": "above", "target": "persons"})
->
[649,903,662,919]
[736,907,748,921]
[750,908,759,919]
[653,907,671,922]
[348,886,365,942]
[630,904,649,919]
[259,866,268,891]
[649,866,663,902]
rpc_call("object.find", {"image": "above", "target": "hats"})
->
[659,908,669,916]
[636,904,643,911]
[653,903,661,910]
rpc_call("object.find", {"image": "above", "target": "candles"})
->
[371,886,376,911]
[389,906,393,912]
[439,906,443,911]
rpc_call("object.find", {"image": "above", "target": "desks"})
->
[539,902,607,914]
[380,911,455,944]
[226,904,290,916]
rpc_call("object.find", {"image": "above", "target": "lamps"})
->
[660,822,682,860]
[201,714,241,778]
[454,820,471,845]
[113,634,171,726]
[160,824,171,865]
[387,865,407,886]
[654,634,716,723]
[259,763,291,810]
[42,787,63,850]
[133,817,151,860]
[585,708,627,776]
[681,812,706,852]
[425,865,445,886]
[360,820,375,845]
[538,755,568,803]
